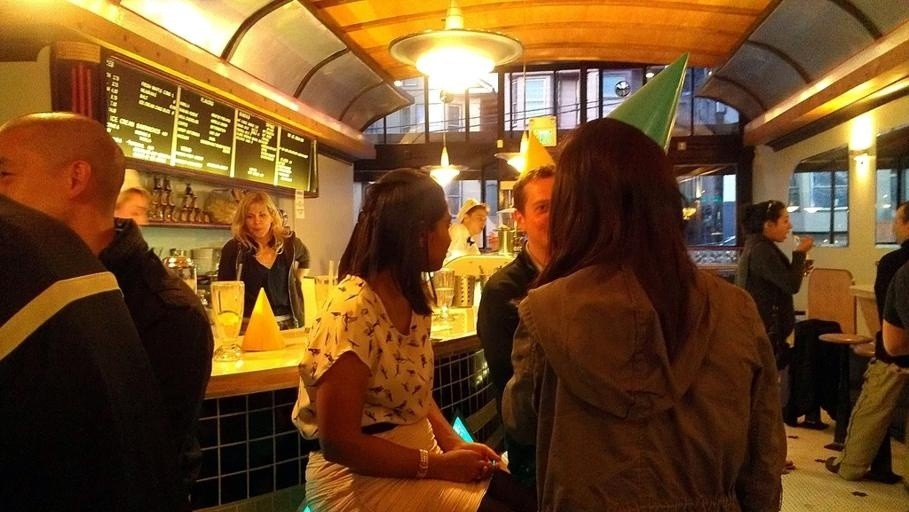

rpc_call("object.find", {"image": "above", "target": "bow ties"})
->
[466,237,475,247]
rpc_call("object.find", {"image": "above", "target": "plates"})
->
[192,249,213,275]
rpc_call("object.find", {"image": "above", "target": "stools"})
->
[819,334,876,451]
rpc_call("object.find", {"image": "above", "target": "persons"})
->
[217,190,308,336]
[881,262,909,358]
[292,169,534,511]
[1,112,213,445]
[733,199,814,475]
[824,203,908,483]
[502,117,787,512]
[476,164,557,451]
[442,199,489,267]
[2,195,142,510]
[110,169,148,229]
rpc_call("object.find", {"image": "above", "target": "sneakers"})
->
[826,456,842,474]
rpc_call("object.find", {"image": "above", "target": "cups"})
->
[197,289,206,308]
[479,273,490,292]
[329,260,339,295]
[314,275,339,310]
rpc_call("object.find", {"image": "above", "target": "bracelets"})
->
[415,448,428,480]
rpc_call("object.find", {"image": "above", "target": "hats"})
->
[519,128,556,181]
[605,51,690,156]
[119,168,148,193]
[455,198,489,224]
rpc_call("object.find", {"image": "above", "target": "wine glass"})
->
[434,269,454,323]
[212,282,245,362]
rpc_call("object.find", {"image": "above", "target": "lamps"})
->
[388,1,524,92]
[421,93,468,191]
[495,63,535,175]
